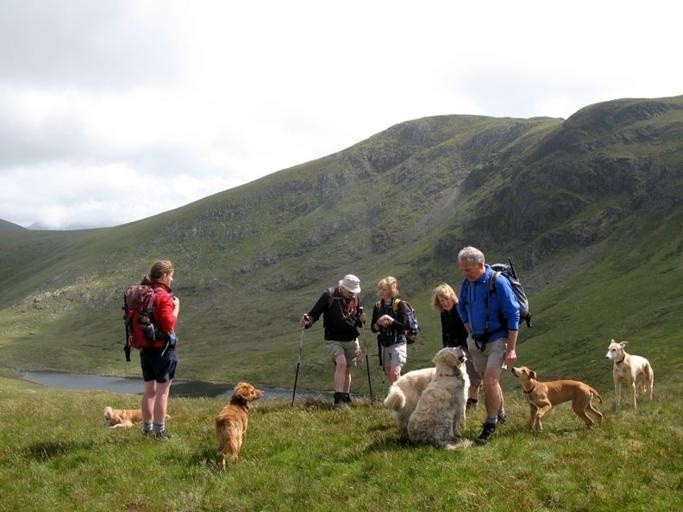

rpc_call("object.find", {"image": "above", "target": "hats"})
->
[339,274,363,294]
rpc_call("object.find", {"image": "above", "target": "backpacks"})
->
[122,283,173,350]
[376,298,418,344]
[464,263,529,326]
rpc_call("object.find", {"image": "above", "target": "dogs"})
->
[605,338,654,411]
[404,345,474,451]
[214,381,264,473]
[103,405,172,430]
[510,364,605,431]
[381,366,437,438]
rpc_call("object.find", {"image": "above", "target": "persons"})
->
[433,283,483,406]
[139,259,180,439]
[300,274,367,405]
[371,276,419,385]
[457,246,520,444]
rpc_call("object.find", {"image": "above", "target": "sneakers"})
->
[478,422,495,441]
[144,428,171,442]
[498,415,507,424]
[333,391,352,404]
[468,398,478,406]
